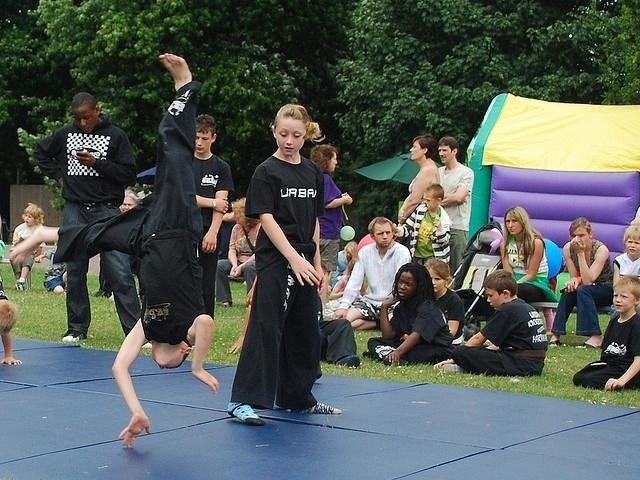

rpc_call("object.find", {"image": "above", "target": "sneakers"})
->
[442,363,461,374]
[62,330,84,343]
[141,342,152,349]
[15,282,23,291]
[375,345,396,359]
[335,355,360,367]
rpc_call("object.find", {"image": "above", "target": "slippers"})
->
[575,342,601,349]
[549,340,560,348]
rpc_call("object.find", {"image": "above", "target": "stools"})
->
[228,276,244,280]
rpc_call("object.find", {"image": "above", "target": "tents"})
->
[460,92,640,299]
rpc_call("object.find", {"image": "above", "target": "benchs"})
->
[527,302,610,312]
[1,259,39,289]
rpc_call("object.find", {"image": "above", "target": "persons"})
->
[225,103,342,428]
[8,53,218,449]
[434,207,553,377]
[214,197,263,306]
[1,236,23,366]
[332,217,466,366]
[10,203,69,293]
[310,145,353,318]
[191,114,235,319]
[33,94,152,350]
[393,134,476,282]
[551,214,639,391]
